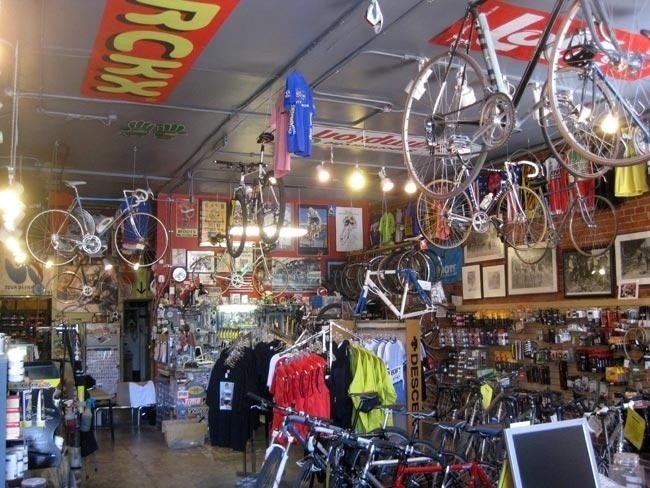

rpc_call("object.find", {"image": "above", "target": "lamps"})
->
[404,62,594,126]
[312,161,419,196]
[0,40,30,271]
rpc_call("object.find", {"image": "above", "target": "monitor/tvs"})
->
[503,418,602,488]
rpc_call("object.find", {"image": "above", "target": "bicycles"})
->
[190,130,292,301]
[26,179,170,306]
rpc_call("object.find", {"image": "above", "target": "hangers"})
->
[227,312,407,383]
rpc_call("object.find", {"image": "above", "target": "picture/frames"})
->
[459,231,650,300]
